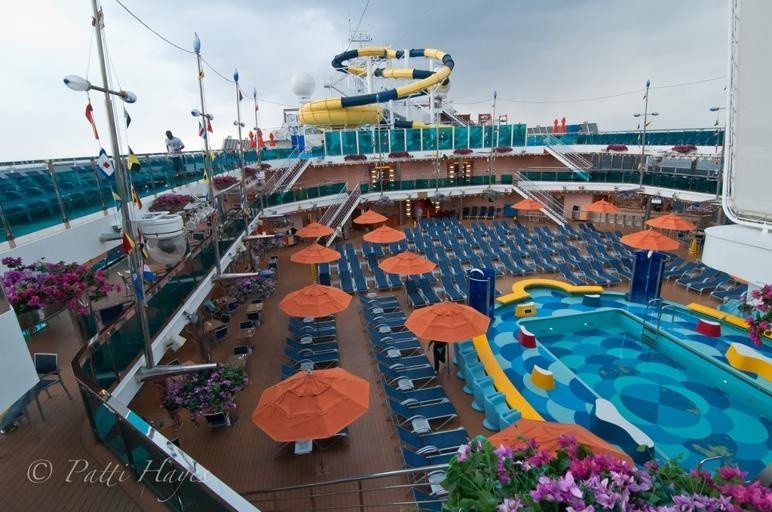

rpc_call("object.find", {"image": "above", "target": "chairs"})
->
[167,250,281,370]
[33,349,69,419]
[281,313,350,446]
[353,292,472,512]
[661,254,748,304]
[315,205,636,308]
[116,271,138,302]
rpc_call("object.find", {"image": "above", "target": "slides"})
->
[298,46,455,128]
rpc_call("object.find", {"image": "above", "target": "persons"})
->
[428,340,447,374]
[416,206,423,225]
[165,131,184,177]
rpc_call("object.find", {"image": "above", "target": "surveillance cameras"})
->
[138,214,186,265]
[254,172,266,191]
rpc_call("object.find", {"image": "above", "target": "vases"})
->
[203,404,233,428]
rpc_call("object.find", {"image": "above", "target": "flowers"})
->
[2,256,126,317]
[670,143,699,155]
[737,280,771,348]
[209,161,272,188]
[157,365,253,416]
[605,144,629,152]
[443,430,772,511]
[343,154,368,162]
[150,193,197,212]
[491,146,514,154]
[453,148,474,156]
[389,151,414,159]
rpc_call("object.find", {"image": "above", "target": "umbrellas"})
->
[353,208,389,230]
[510,197,544,224]
[378,249,437,303]
[253,368,369,443]
[249,130,275,148]
[279,281,352,339]
[363,223,406,257]
[586,198,620,229]
[553,119,558,133]
[290,242,341,281]
[619,227,680,251]
[295,223,335,241]
[561,117,566,132]
[646,211,697,237]
[482,418,634,473]
[404,301,491,375]
